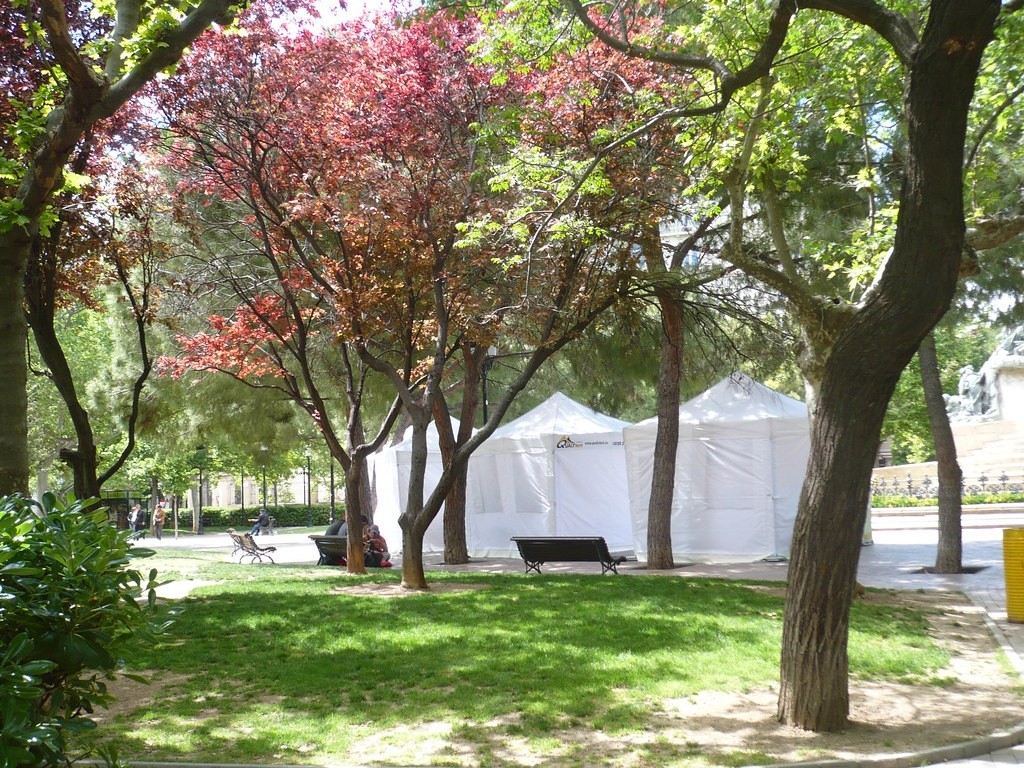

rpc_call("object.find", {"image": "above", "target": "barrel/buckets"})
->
[1003,528,1024,624]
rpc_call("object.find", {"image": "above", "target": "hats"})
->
[368,526,380,536]
[260,509,266,513]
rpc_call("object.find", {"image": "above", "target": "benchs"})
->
[260,517,276,536]
[511,536,627,574]
[226,529,277,564]
[308,534,383,566]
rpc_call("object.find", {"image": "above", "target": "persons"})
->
[251,509,269,536]
[324,513,393,568]
[127,504,146,541]
[153,504,165,540]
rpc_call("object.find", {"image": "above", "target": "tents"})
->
[623,371,874,565]
[350,390,634,560]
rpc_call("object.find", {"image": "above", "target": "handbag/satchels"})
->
[364,549,382,567]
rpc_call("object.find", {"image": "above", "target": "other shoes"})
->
[381,560,392,566]
